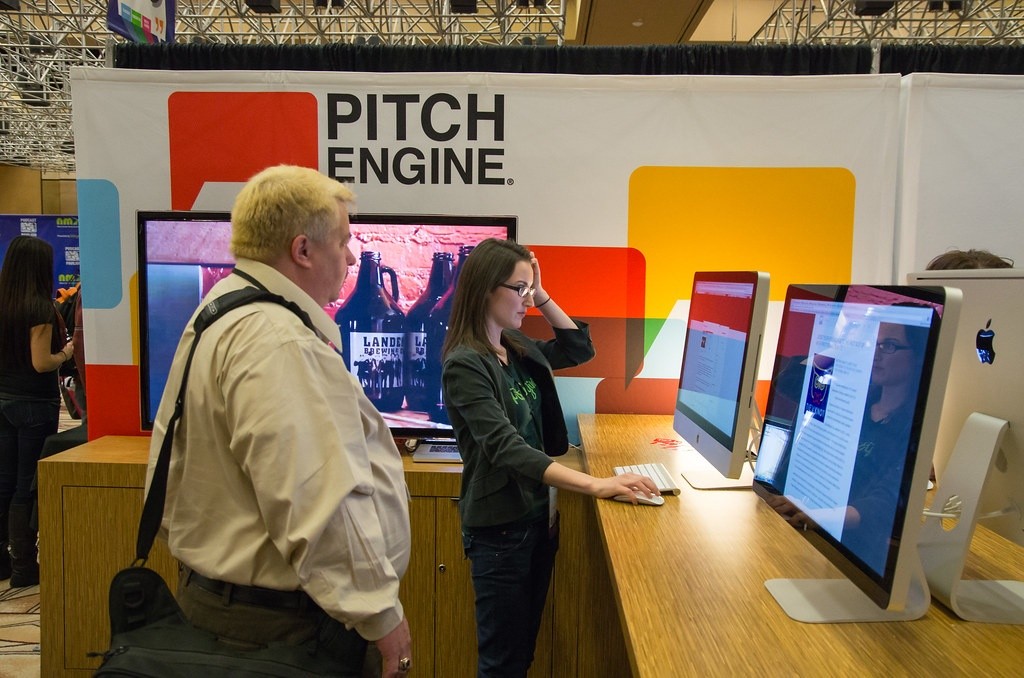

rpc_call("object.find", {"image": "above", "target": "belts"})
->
[178,561,323,610]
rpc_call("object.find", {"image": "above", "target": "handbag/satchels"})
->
[92,567,370,677]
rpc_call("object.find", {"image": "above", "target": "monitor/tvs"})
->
[137,211,518,440]
[674,271,1024,625]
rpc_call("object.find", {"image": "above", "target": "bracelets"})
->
[59,350,67,362]
[536,297,551,309]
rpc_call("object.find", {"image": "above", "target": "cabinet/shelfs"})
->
[38,436,633,678]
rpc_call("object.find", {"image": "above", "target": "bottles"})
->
[334,251,408,412]
[404,254,456,411]
[424,246,481,424]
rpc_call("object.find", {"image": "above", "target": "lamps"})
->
[245,0,282,13]
[534,0,546,8]
[855,0,894,15]
[0,0,20,11]
[929,0,964,12]
[516,0,530,9]
[315,0,345,9]
[450,0,479,13]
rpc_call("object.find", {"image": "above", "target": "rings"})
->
[399,657,410,670]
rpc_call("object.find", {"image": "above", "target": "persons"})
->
[777,301,941,542]
[352,350,401,388]
[145,166,413,678]
[441,238,661,678]
[926,248,1013,272]
[0,235,88,587]
[410,351,425,386]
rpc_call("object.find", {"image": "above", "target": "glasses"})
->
[497,281,536,298]
[877,343,915,354]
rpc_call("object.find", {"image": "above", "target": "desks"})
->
[576,411,1024,677]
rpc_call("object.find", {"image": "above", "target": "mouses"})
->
[613,491,664,505]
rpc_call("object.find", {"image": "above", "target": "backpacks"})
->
[54,281,81,377]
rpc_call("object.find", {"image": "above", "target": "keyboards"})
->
[614,463,681,495]
[413,443,463,463]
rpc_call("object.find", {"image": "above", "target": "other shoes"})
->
[10,563,39,587]
[0,556,11,580]
